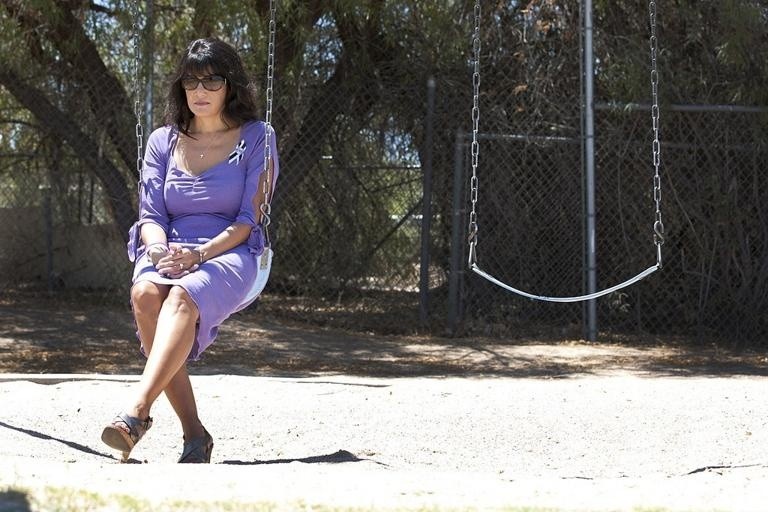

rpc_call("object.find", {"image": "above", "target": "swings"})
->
[130,0,277,315]
[468,0,665,303]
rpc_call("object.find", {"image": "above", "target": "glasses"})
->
[180,73,227,92]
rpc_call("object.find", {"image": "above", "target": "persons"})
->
[102,37,280,462]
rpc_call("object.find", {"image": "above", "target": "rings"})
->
[180,262,184,270]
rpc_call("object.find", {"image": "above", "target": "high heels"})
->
[100,410,154,464]
[175,426,214,463]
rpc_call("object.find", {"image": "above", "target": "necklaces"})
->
[188,131,221,160]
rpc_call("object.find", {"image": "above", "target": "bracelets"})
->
[195,246,204,264]
